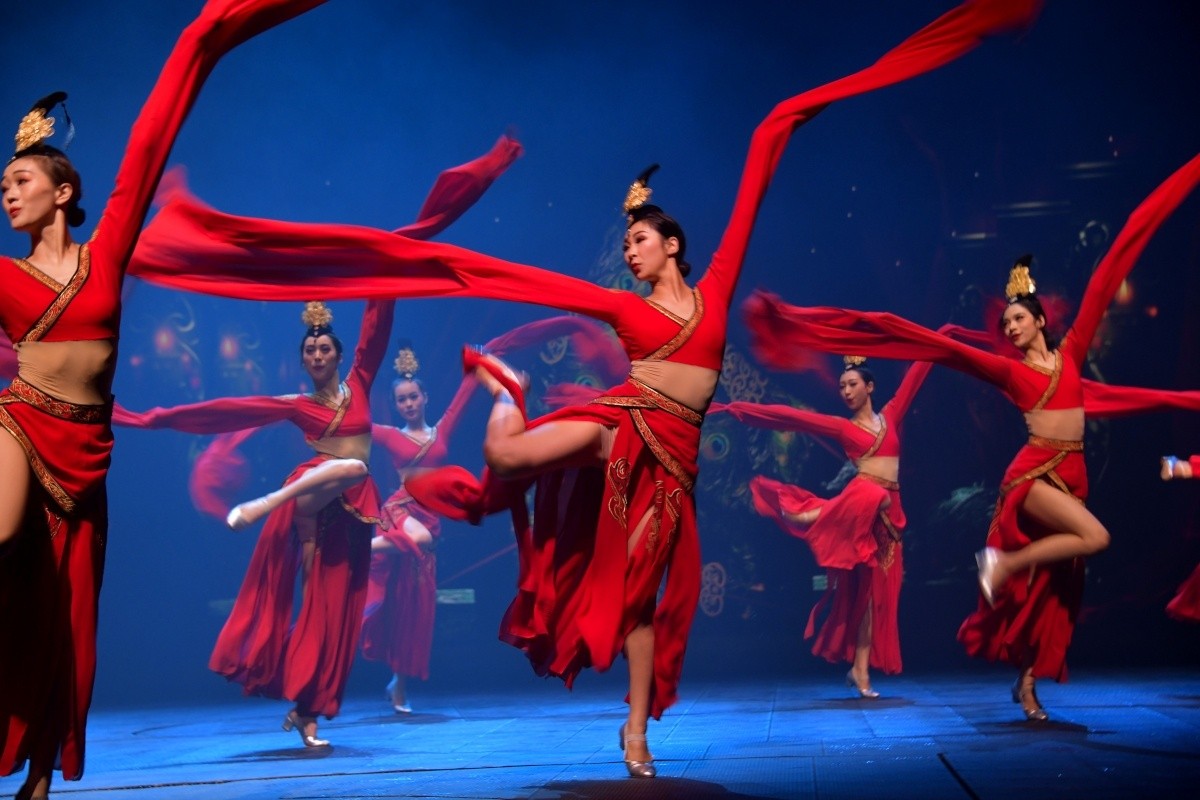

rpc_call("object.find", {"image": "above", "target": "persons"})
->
[465,0,1033,781]
[0,0,332,800]
[704,354,934,700]
[110,133,524,745]
[740,150,1200,722]
[1160,454,1200,620]
[192,314,631,714]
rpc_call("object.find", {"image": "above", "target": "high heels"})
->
[283,710,330,749]
[976,547,999,606]
[619,722,656,777]
[846,670,879,699]
[385,686,413,714]
[1011,681,1047,721]
[226,496,271,531]
[467,343,531,394]
[1161,456,1185,481]
